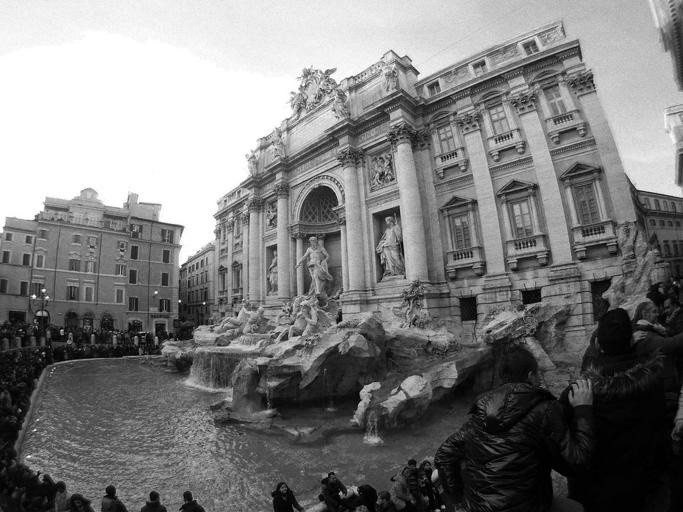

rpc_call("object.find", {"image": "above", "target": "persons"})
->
[376,216,403,277]
[267,250,278,295]
[294,237,332,296]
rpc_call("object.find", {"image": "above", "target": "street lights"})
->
[30,287,49,334]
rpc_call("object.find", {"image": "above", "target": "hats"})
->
[596,307,634,357]
